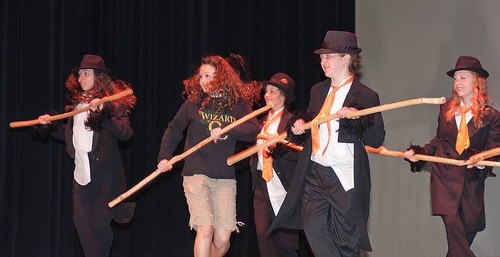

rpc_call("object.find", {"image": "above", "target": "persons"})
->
[250,71,306,257]
[158,55,261,257]
[402,55,500,257]
[38,54,133,257]
[300,30,386,256]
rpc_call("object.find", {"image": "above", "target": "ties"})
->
[261,109,284,182]
[311,76,354,157]
[456,106,472,156]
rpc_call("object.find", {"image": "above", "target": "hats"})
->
[72,54,112,72]
[262,73,296,104]
[446,56,489,80]
[314,31,362,54]
